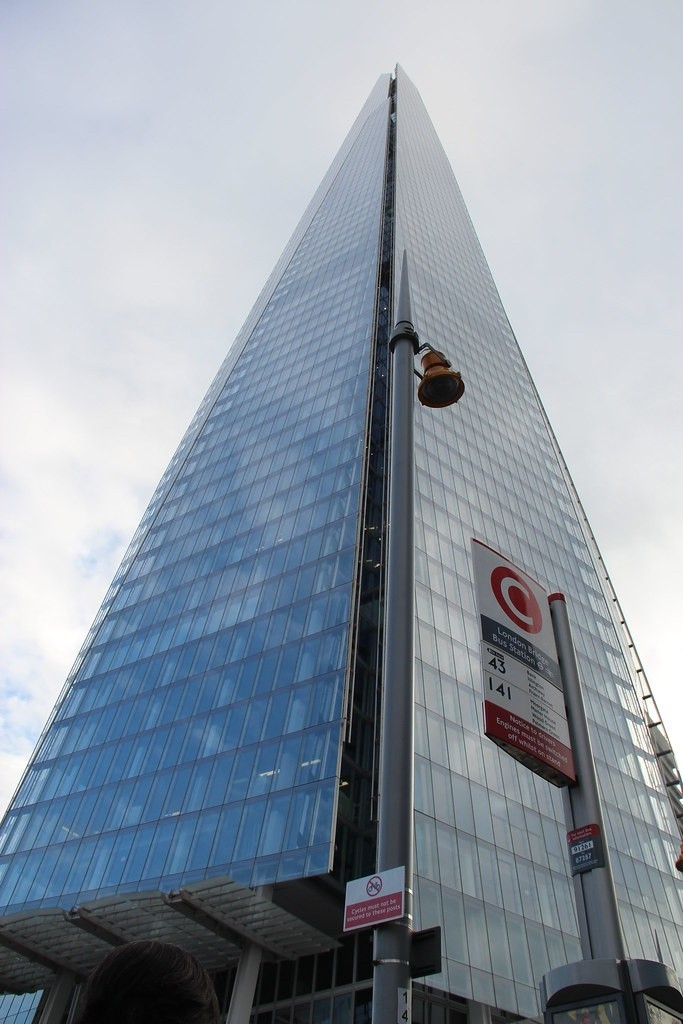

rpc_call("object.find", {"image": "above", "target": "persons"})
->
[74,940,223,1024]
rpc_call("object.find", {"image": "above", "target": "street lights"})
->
[371,249,465,1024]
